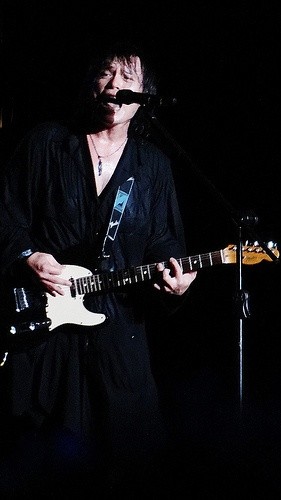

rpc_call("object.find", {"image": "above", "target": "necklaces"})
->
[89,134,128,176]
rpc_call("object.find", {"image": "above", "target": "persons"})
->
[0,36,198,500]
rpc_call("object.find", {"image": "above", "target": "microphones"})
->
[116,89,178,109]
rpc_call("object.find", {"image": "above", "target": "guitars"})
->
[0,238,280,352]
